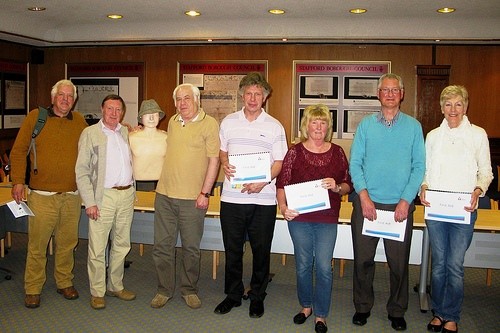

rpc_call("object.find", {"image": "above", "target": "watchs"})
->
[336,184,342,193]
[201,191,210,198]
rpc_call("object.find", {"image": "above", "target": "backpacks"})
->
[7,105,46,185]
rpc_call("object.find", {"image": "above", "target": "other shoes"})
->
[293,307,312,324]
[427,316,445,332]
[315,321,328,333]
[442,322,458,333]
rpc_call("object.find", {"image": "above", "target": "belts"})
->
[113,184,132,190]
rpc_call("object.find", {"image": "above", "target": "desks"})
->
[0,188,500,286]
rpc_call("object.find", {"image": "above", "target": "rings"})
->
[288,216,290,219]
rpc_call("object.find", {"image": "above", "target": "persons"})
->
[150,84,220,308]
[74,94,137,309]
[211,74,290,318]
[277,104,352,333]
[347,73,425,331]
[419,85,493,333]
[10,80,134,310]
[128,99,172,192]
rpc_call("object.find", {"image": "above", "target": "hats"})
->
[137,99,165,124]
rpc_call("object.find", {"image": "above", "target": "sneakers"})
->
[151,293,173,308]
[91,294,106,309]
[353,311,371,326]
[106,288,136,300]
[387,314,406,330]
[181,294,201,309]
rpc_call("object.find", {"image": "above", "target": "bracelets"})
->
[268,182,271,184]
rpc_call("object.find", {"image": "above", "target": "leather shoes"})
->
[214,296,242,315]
[56,286,79,300]
[24,294,40,308]
[249,301,264,318]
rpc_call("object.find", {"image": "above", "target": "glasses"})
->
[380,88,401,94]
